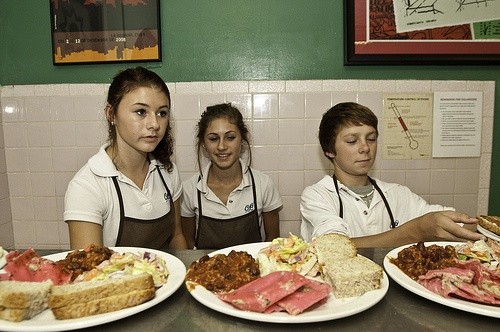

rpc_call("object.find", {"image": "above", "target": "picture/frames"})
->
[343,0,500,65]
[49,0,161,67]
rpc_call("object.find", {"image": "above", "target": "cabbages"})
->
[270,232,313,266]
[91,254,169,290]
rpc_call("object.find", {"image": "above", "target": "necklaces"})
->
[359,186,374,197]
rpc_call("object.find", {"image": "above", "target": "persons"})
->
[300,102,487,249]
[179,103,283,249]
[63,67,187,250]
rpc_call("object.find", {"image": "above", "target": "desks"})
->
[0,248,500,332]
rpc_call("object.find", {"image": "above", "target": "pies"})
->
[257,253,318,277]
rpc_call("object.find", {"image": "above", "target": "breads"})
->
[48,272,155,320]
[0,279,55,322]
[478,214,500,236]
[314,233,384,299]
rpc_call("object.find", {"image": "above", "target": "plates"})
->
[477,225,500,242]
[185,240,390,323]
[383,242,500,319]
[0,246,187,332]
